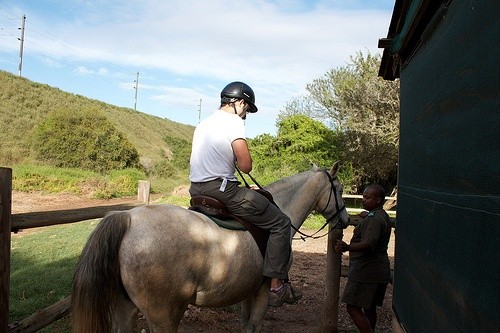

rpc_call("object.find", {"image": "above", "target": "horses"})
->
[70,160,351,333]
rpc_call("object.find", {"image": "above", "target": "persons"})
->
[189,80,303,308]
[334,183,392,332]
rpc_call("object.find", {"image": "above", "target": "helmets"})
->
[221,81,258,113]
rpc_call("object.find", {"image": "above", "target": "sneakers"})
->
[268,282,302,307]
